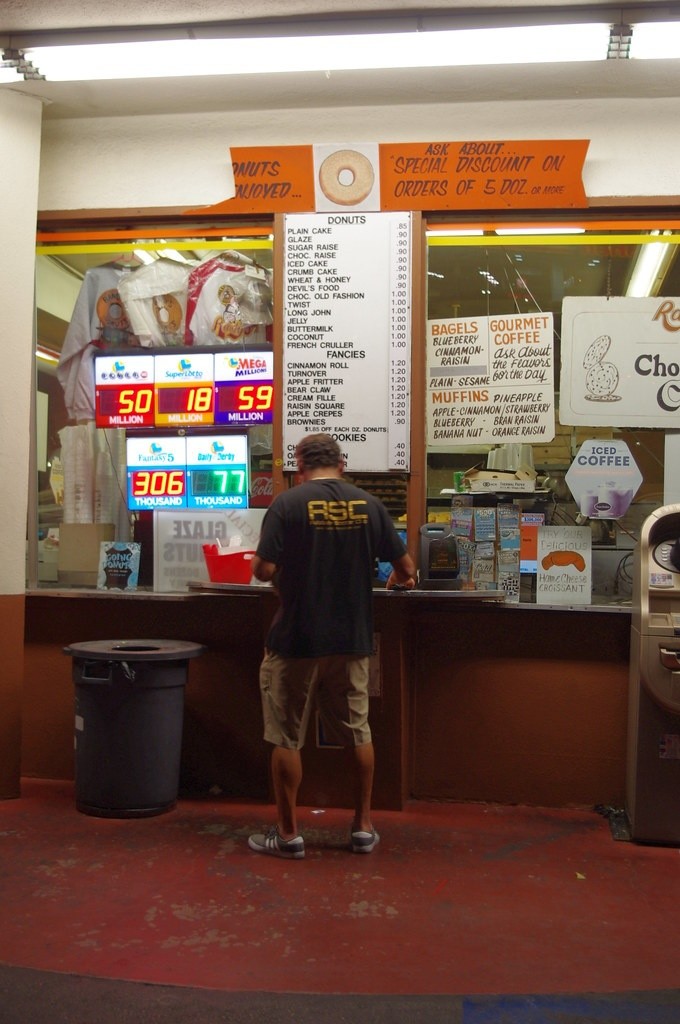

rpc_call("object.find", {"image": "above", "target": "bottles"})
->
[453,471,465,492]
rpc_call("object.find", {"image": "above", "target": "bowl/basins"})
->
[202,545,256,584]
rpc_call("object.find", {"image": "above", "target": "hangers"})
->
[217,250,271,275]
[155,238,188,266]
[113,252,143,265]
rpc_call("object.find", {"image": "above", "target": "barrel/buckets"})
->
[70,658,189,820]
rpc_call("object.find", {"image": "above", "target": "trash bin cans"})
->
[60,641,209,818]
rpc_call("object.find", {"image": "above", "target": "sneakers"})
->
[350,825,381,852]
[248,834,306,859]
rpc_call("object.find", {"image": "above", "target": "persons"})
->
[248,432,416,860]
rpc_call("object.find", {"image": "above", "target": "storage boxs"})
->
[470,478,536,491]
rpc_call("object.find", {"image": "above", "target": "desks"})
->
[438,488,548,504]
